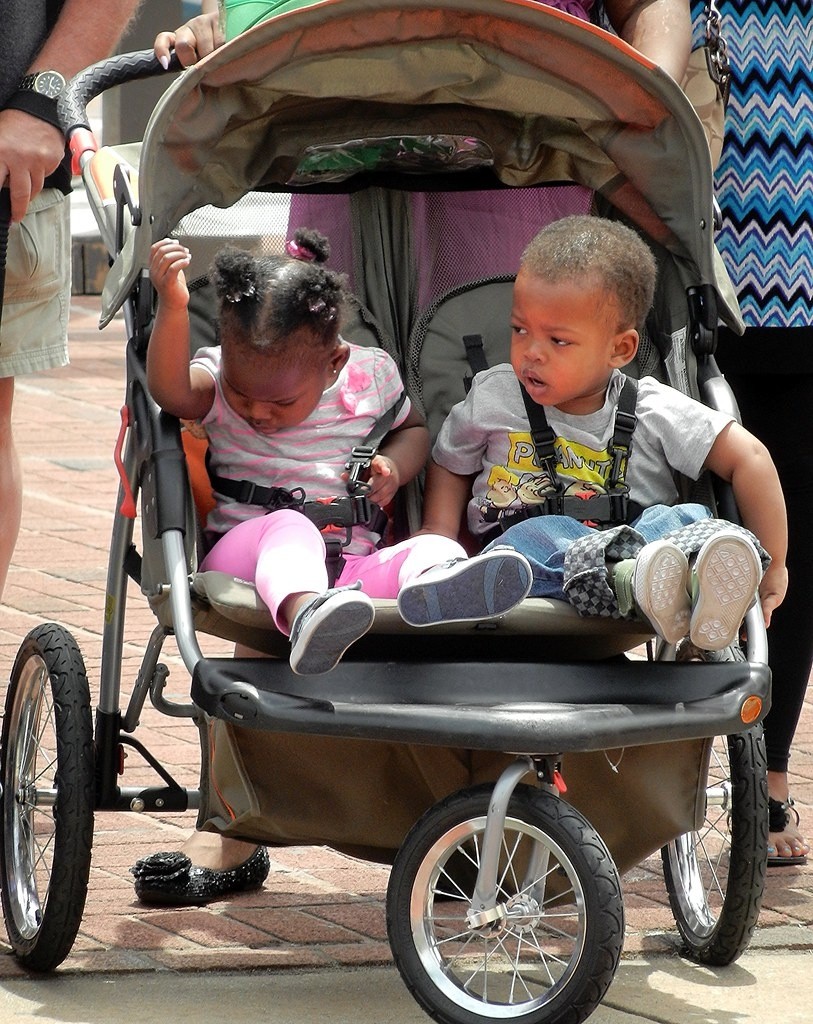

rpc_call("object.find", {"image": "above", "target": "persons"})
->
[690,0,813,864]
[0,1,141,602]
[131,1,691,905]
[145,224,533,671]
[412,216,788,649]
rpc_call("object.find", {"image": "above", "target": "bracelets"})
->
[0,91,63,133]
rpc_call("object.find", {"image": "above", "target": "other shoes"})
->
[128,846,270,906]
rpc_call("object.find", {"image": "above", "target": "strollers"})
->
[0,0,772,1024]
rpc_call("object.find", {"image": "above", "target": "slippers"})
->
[767,796,808,865]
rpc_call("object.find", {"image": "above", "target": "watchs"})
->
[18,70,65,99]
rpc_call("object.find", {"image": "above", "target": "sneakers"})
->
[688,530,763,652]
[288,581,376,677]
[612,541,692,645]
[397,544,533,626]
